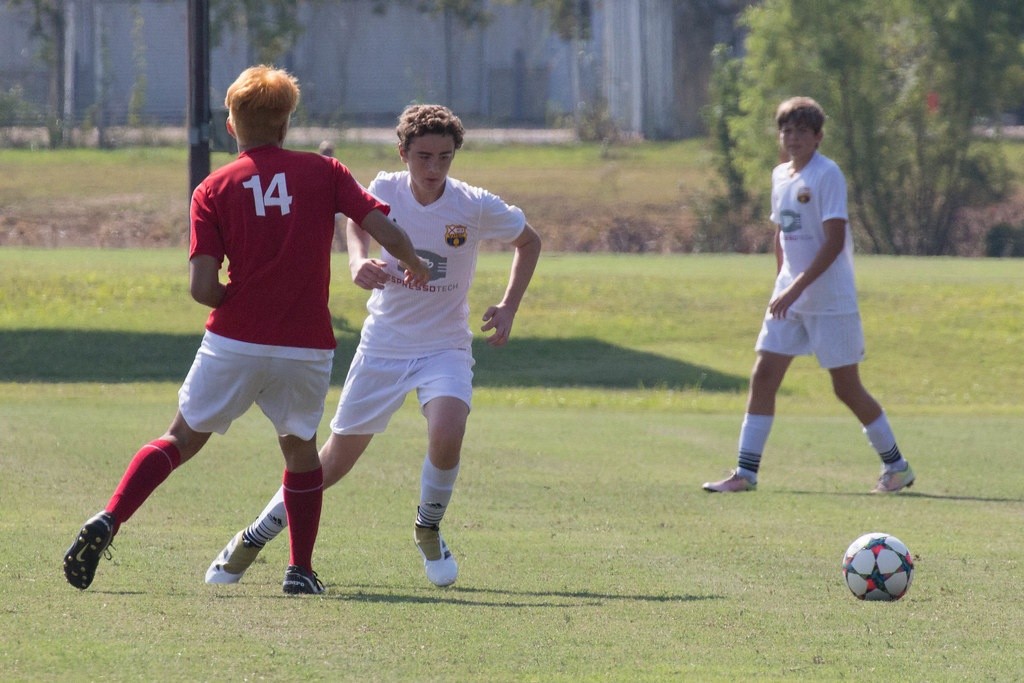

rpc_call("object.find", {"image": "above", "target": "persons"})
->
[61,65,428,594]
[702,98,914,493]
[204,104,541,587]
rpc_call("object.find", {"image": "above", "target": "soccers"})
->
[842,532,915,602]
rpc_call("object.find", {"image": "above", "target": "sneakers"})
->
[281,565,324,595]
[869,459,915,495]
[702,469,757,492]
[63,510,116,589]
[413,525,458,587]
[204,529,263,583]
